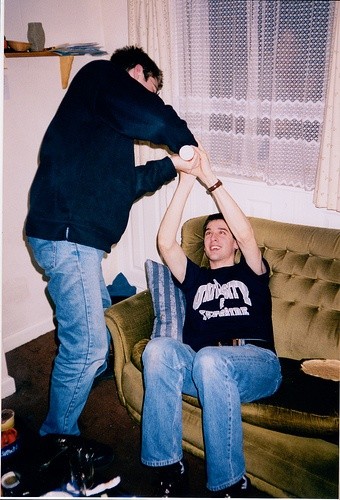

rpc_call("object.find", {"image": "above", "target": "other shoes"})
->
[152,462,190,498]
[53,437,114,468]
[209,476,253,498]
[92,356,116,388]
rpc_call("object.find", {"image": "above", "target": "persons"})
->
[25,45,200,435]
[140,144,282,498]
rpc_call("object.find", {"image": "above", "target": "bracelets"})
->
[207,180,222,193]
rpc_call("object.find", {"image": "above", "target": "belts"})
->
[218,339,278,357]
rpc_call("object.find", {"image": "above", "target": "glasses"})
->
[151,80,162,97]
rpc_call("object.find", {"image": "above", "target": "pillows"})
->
[145,259,185,344]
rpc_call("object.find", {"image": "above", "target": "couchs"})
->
[104,214,338,499]
